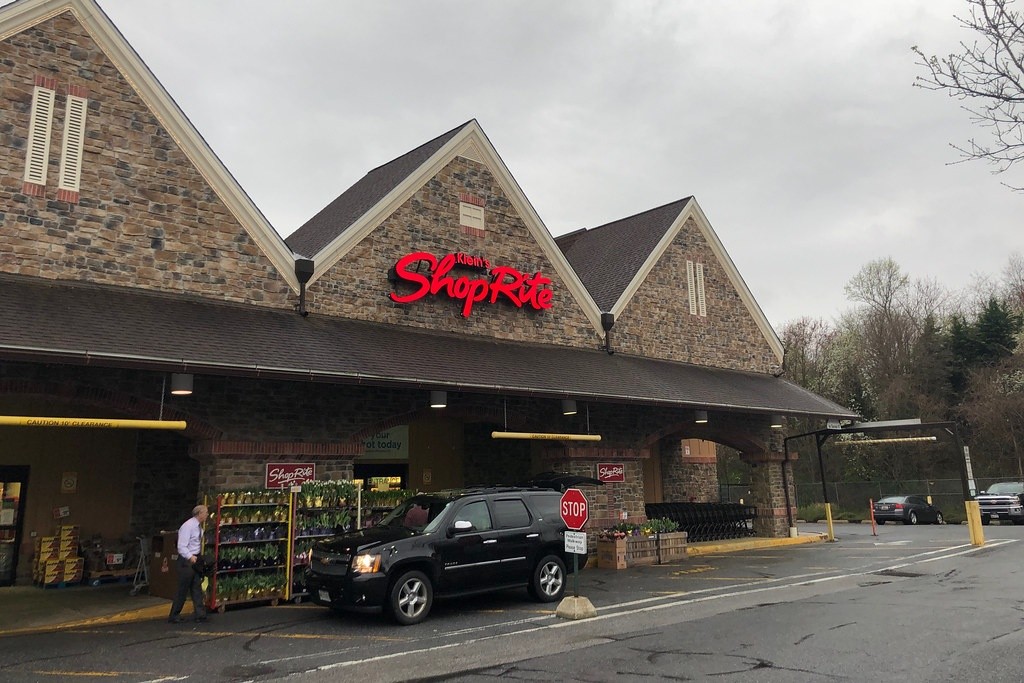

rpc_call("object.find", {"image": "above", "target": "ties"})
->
[198,524,202,554]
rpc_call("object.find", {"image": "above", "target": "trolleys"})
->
[129,534,161,597]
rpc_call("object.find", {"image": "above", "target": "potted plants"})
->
[294,478,357,586]
[362,489,416,528]
[211,490,286,596]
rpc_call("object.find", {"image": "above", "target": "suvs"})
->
[974,481,1024,525]
[300,470,607,624]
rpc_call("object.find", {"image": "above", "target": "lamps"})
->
[170,373,194,395]
[429,392,448,408]
[562,401,577,415]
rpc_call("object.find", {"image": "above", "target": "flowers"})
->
[595,519,689,538]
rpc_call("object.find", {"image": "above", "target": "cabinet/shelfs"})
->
[292,482,361,603]
[206,489,292,612]
[361,487,419,531]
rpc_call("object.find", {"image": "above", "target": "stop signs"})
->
[559,487,590,530]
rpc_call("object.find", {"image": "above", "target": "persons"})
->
[167,504,214,622]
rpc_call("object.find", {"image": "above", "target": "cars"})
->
[872,494,944,525]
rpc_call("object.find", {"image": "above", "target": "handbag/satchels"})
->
[191,555,217,585]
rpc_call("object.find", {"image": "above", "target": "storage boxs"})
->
[32,526,84,586]
[105,552,123,565]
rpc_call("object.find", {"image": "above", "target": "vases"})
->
[597,538,627,570]
[657,533,689,563]
[628,535,658,565]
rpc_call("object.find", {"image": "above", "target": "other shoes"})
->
[196,614,214,622]
[168,615,188,622]
